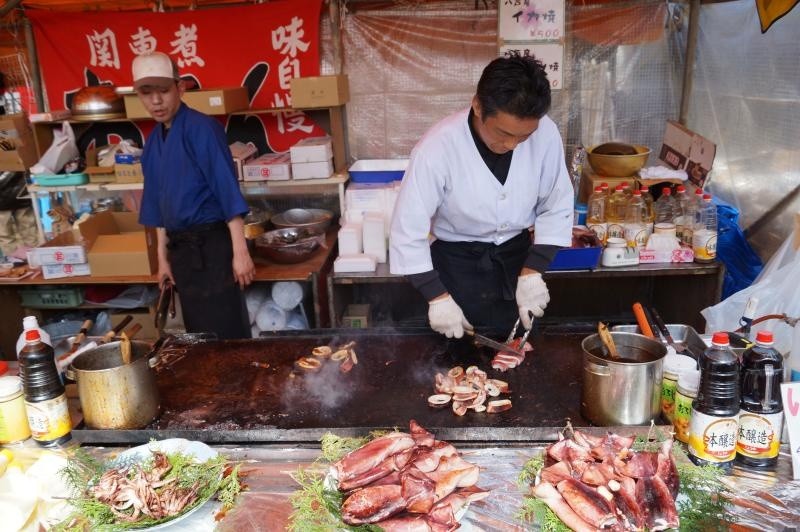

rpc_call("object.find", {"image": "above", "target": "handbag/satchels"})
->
[698,227,800,384]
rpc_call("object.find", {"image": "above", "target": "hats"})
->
[131,51,181,90]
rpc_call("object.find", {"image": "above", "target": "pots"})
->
[65,338,164,429]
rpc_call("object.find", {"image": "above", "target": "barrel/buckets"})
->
[581,332,669,427]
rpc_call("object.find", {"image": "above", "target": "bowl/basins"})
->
[254,226,325,265]
[271,208,334,230]
[585,142,653,179]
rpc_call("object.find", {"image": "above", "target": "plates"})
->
[106,437,224,532]
[535,463,545,486]
[322,446,471,532]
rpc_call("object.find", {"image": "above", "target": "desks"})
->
[325,262,725,346]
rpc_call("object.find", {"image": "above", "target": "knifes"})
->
[464,329,523,357]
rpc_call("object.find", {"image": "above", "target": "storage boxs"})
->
[543,225,602,272]
[343,304,372,329]
[579,168,635,207]
[0,73,414,341]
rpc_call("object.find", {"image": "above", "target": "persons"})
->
[132,52,255,339]
[389,49,574,338]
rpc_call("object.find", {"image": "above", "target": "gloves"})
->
[428,293,473,339]
[515,273,551,331]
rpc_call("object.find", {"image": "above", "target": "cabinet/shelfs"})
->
[0,106,363,342]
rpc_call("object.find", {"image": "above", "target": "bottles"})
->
[661,354,701,446]
[573,182,718,260]
[16,316,63,384]
[686,330,784,474]
[0,376,30,444]
[18,330,73,446]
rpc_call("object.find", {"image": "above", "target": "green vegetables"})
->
[278,427,407,532]
[515,430,741,532]
[50,447,244,532]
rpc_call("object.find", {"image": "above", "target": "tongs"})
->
[505,312,534,350]
[154,279,176,329]
